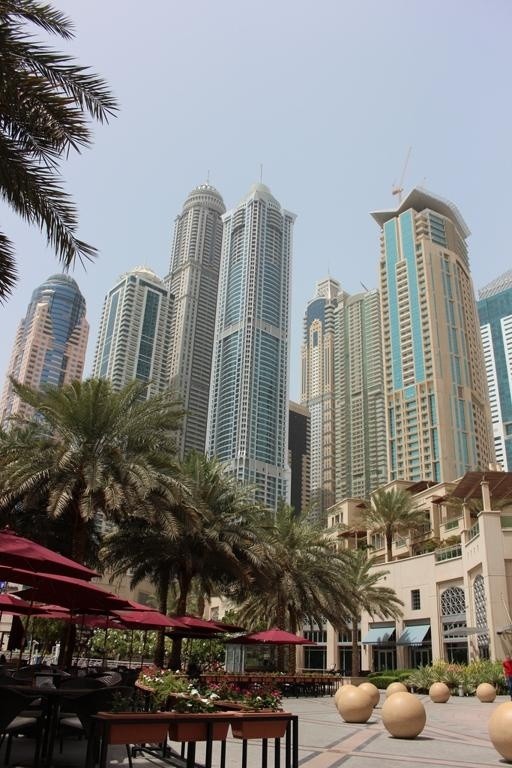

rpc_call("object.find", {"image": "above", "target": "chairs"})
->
[216,673,343,700]
[2,655,139,765]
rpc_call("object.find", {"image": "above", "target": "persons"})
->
[502,652,512,701]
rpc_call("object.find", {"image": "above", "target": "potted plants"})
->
[406,658,463,698]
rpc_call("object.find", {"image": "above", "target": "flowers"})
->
[218,681,280,711]
[143,662,215,711]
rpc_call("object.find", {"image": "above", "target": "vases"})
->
[98,707,291,746]
